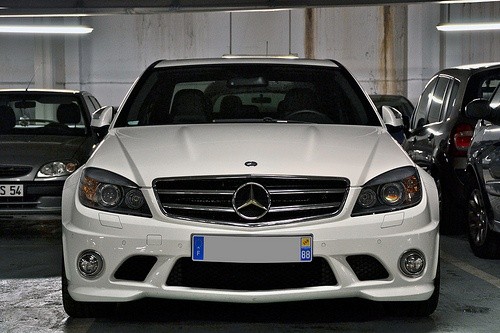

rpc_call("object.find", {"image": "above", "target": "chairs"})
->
[0,105,23,135]
[276,88,330,114]
[160,89,214,125]
[57,103,85,136]
[220,96,242,113]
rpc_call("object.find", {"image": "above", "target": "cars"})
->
[464,78,500,258]
[369,94,415,144]
[1,87,100,225]
[400,63,500,233]
[60,54,440,317]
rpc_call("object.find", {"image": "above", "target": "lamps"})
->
[435,23,500,32]
[0,24,95,34]
